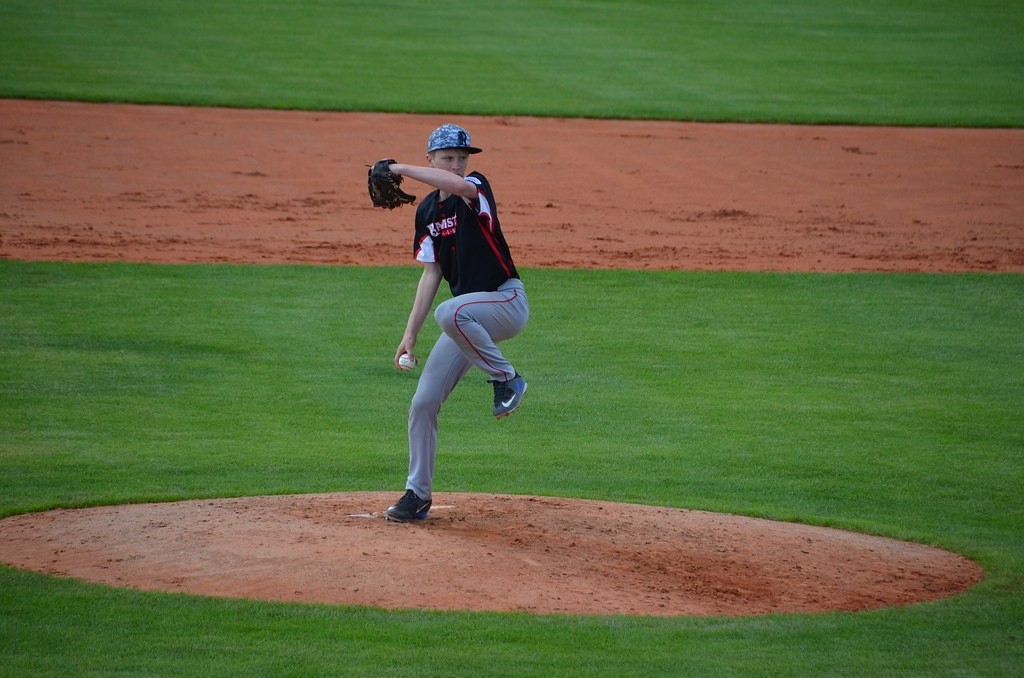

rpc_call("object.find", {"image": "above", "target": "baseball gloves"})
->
[367,155,417,211]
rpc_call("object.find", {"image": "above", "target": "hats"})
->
[427,122,483,154]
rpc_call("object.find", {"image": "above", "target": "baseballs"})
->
[398,353,415,371]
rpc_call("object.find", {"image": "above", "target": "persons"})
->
[367,122,528,523]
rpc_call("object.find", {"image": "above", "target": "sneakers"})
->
[487,371,528,420]
[383,488,432,523]
